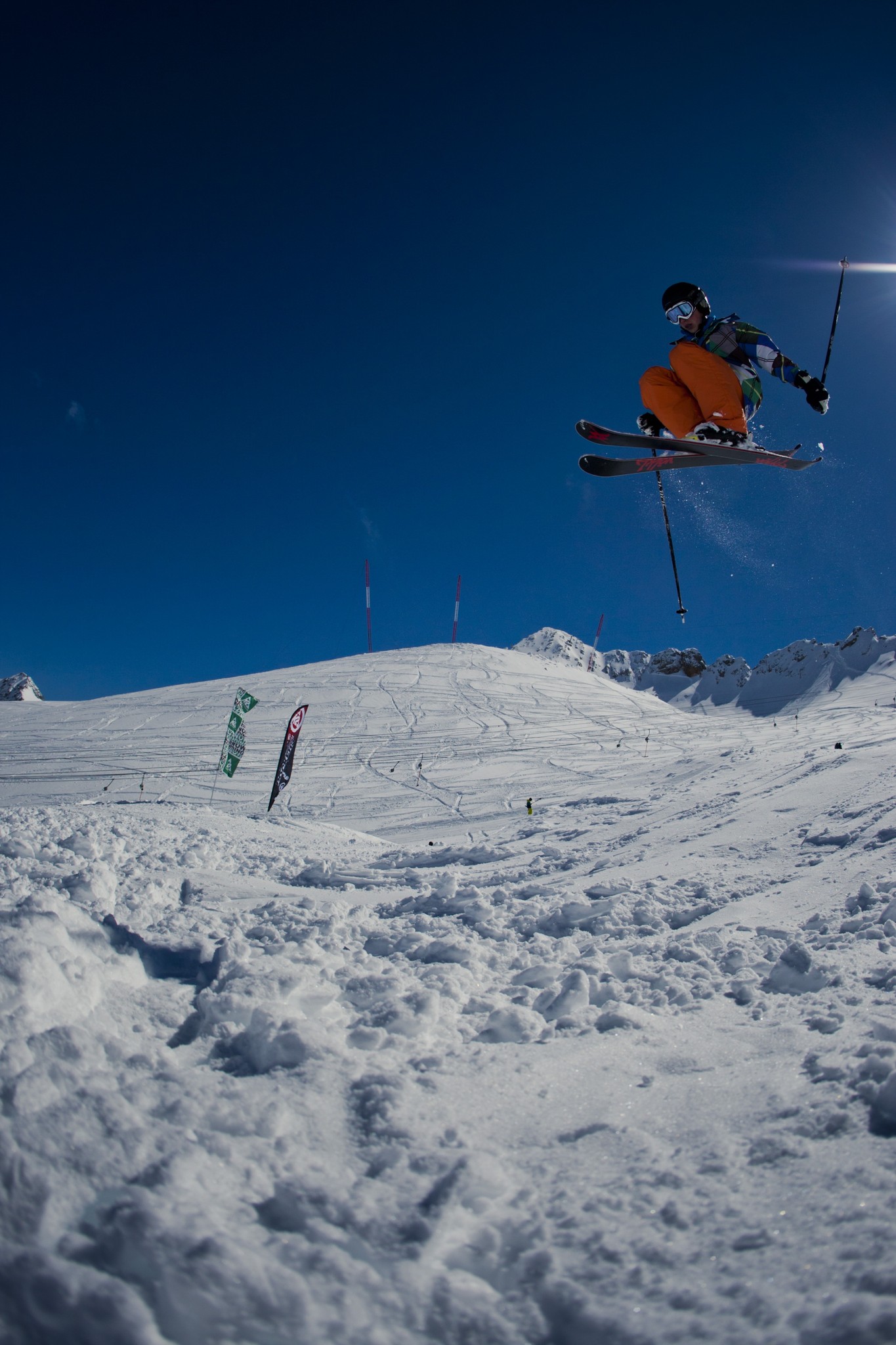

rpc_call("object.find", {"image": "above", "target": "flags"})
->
[218,688,259,778]
[266,703,310,812]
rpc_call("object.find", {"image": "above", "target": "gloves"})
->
[639,412,670,437]
[806,377,830,415]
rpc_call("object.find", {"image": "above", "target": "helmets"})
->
[661,281,711,317]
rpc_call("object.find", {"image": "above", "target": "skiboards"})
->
[575,418,824,478]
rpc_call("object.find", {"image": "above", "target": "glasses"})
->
[664,290,702,324]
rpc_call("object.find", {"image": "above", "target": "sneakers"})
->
[696,422,753,451]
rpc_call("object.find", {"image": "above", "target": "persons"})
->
[638,283,831,445]
